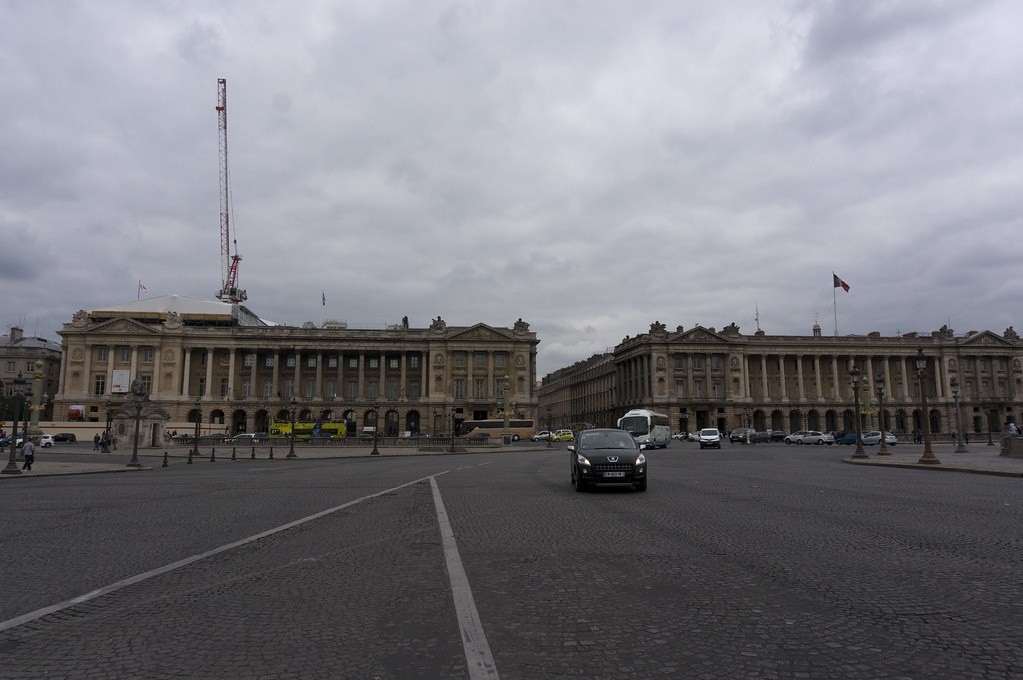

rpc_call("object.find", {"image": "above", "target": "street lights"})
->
[285,396,298,457]
[192,398,202,454]
[0,370,28,475]
[546,407,554,448]
[849,365,869,459]
[451,405,456,452]
[913,344,941,465]
[950,379,969,454]
[369,401,381,456]
[432,408,438,438]
[127,387,146,467]
[983,404,996,446]
[874,373,892,456]
[100,400,112,453]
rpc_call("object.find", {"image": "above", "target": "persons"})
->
[0,430,7,439]
[965,432,969,445]
[723,431,731,437]
[167,430,177,437]
[1001,421,1019,450]
[92,432,106,451]
[913,432,922,444]
[832,432,845,439]
[952,432,956,446]
[685,431,689,439]
[21,438,35,471]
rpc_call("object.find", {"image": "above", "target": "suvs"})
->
[698,428,723,450]
[567,426,648,493]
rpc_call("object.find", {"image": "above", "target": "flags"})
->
[140,282,150,292]
[833,274,850,293]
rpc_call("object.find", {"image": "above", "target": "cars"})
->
[729,426,857,446]
[172,429,266,445]
[0,431,55,448]
[530,428,579,442]
[52,432,76,443]
[354,434,374,443]
[862,431,898,446]
[671,430,701,442]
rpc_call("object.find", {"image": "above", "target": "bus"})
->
[616,408,671,449]
[267,417,348,445]
[456,418,536,442]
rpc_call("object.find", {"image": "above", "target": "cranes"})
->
[215,77,250,306]
